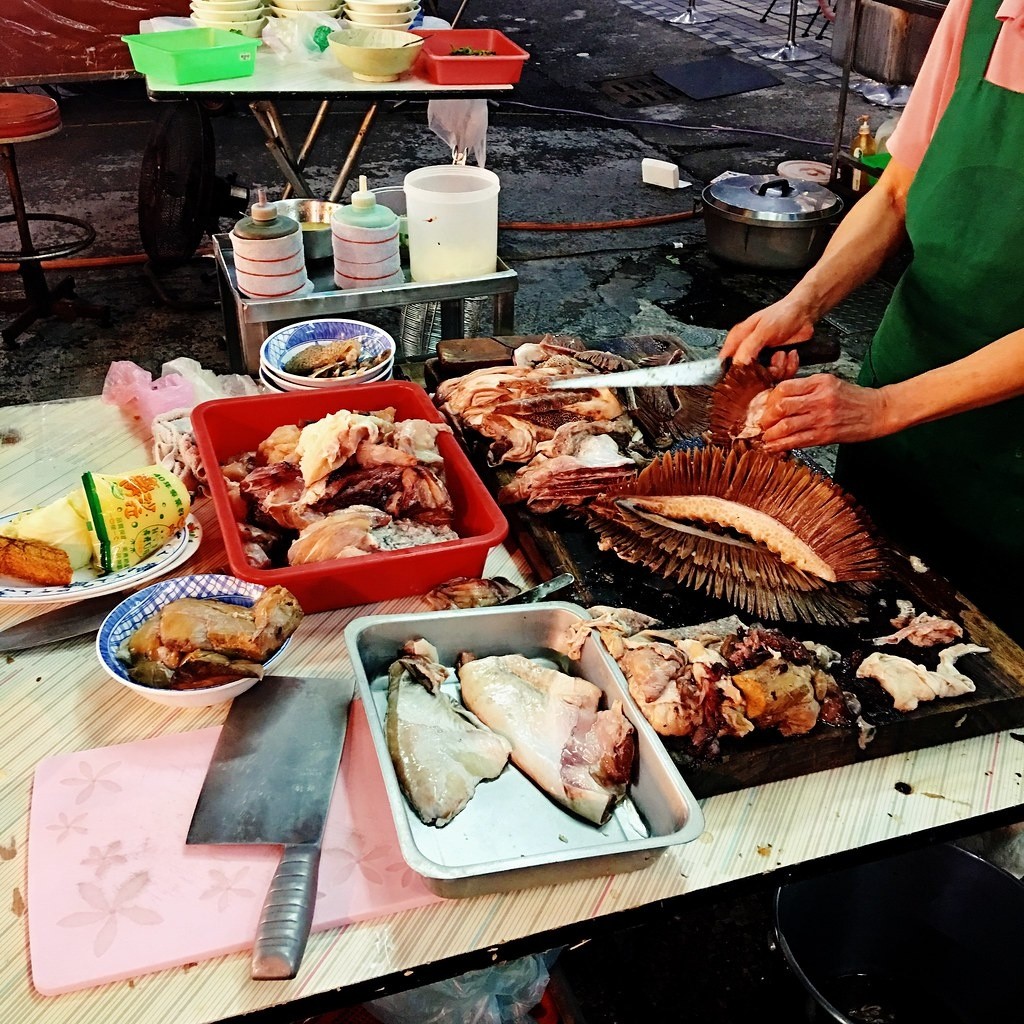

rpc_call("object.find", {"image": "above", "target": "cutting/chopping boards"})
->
[422,331,1024,802]
[27,698,446,998]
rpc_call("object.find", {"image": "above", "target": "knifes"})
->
[182,674,358,983]
[542,337,841,390]
[0,563,232,653]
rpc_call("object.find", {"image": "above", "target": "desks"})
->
[0,375,1023,1024]
[211,232,520,376]
[0,1,213,271]
[138,18,514,202]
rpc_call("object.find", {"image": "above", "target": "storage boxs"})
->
[121,28,263,85]
[191,379,510,615]
[408,29,530,84]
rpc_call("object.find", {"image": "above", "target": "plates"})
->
[0,508,203,604]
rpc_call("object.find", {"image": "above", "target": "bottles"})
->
[230,188,308,299]
[330,174,400,290]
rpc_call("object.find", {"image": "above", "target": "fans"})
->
[139,103,250,308]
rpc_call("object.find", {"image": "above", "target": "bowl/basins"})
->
[270,197,346,259]
[326,29,425,83]
[96,574,294,707]
[268,0,341,25]
[700,174,844,267]
[258,318,396,395]
[342,0,422,29]
[190,0,266,37]
[367,186,407,218]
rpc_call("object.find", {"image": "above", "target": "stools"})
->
[0,92,96,347]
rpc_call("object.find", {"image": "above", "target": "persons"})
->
[720,0,1024,660]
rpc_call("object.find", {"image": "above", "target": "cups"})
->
[402,164,501,282]
